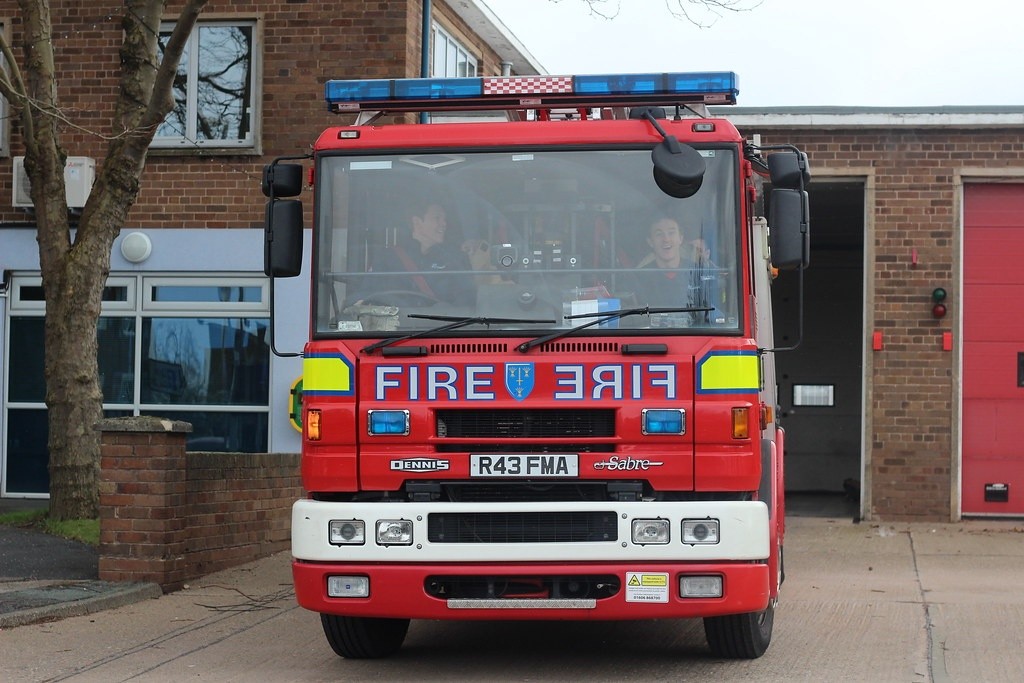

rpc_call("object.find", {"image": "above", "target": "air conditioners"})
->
[11,156,95,208]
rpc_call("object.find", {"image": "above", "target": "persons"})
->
[622,212,725,320]
[363,199,478,317]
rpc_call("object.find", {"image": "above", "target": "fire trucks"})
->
[262,70,813,662]
